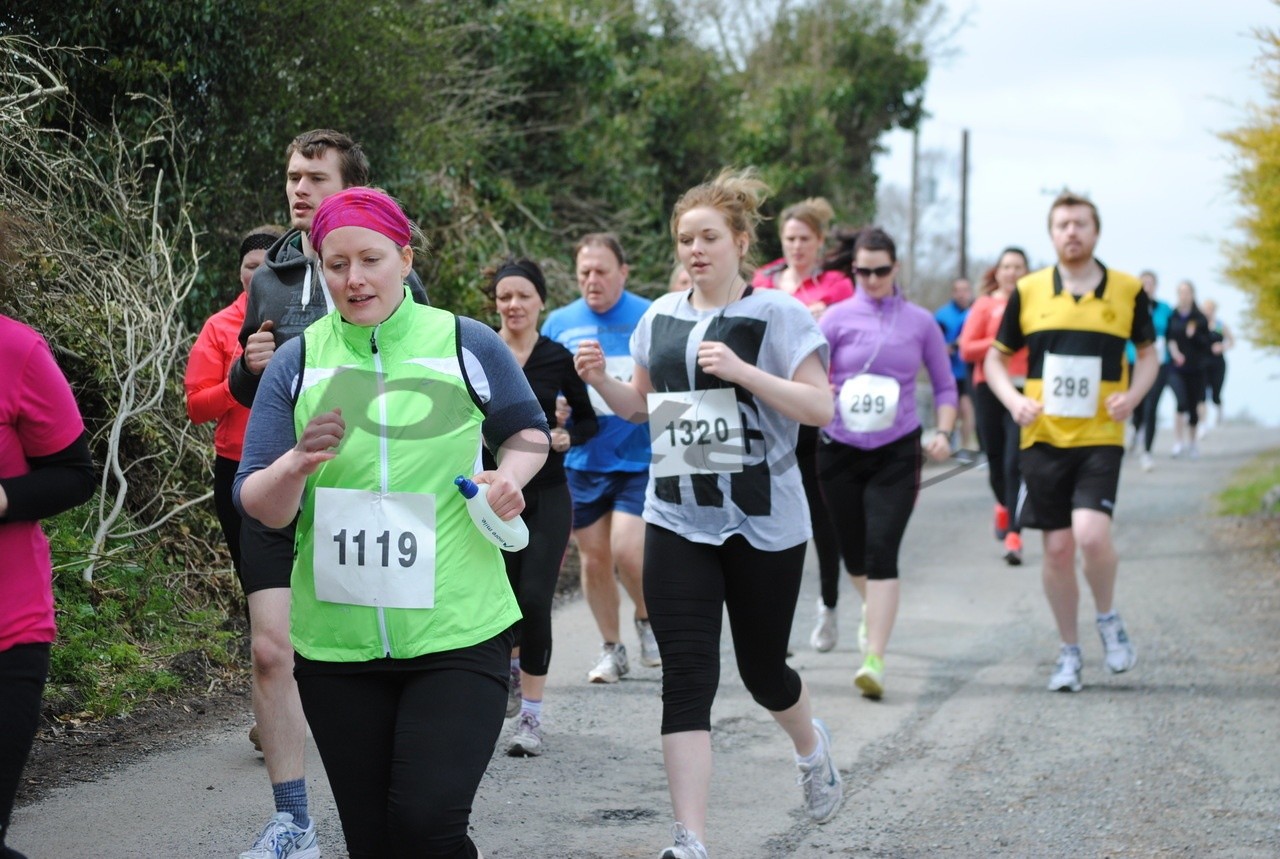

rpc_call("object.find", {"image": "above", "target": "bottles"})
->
[456,474,529,553]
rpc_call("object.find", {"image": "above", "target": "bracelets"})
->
[936,430,950,439]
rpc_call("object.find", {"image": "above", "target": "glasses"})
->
[853,262,893,276]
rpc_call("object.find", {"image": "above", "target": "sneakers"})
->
[1047,646,1082,692]
[993,504,1009,541]
[635,615,662,666]
[811,596,838,652]
[504,666,521,718]
[238,812,321,859]
[1004,532,1021,565]
[660,820,706,859]
[589,642,630,683]
[1097,614,1135,672]
[506,711,542,757]
[794,717,843,823]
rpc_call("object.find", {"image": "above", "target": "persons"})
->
[957,248,1030,563]
[816,223,959,701]
[574,163,844,859]
[1196,297,1234,438]
[983,180,1159,692]
[229,129,434,859]
[234,188,553,859]
[936,277,975,463]
[0,315,99,859]
[753,197,854,654]
[1164,279,1210,461]
[670,263,693,292]
[186,227,289,757]
[479,256,600,758]
[539,233,652,681]
[1126,269,1172,470]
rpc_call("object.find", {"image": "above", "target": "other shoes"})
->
[248,723,262,751]
[1138,450,1154,470]
[856,655,884,700]
[1171,439,1182,458]
[858,604,868,651]
[1123,423,1138,453]
